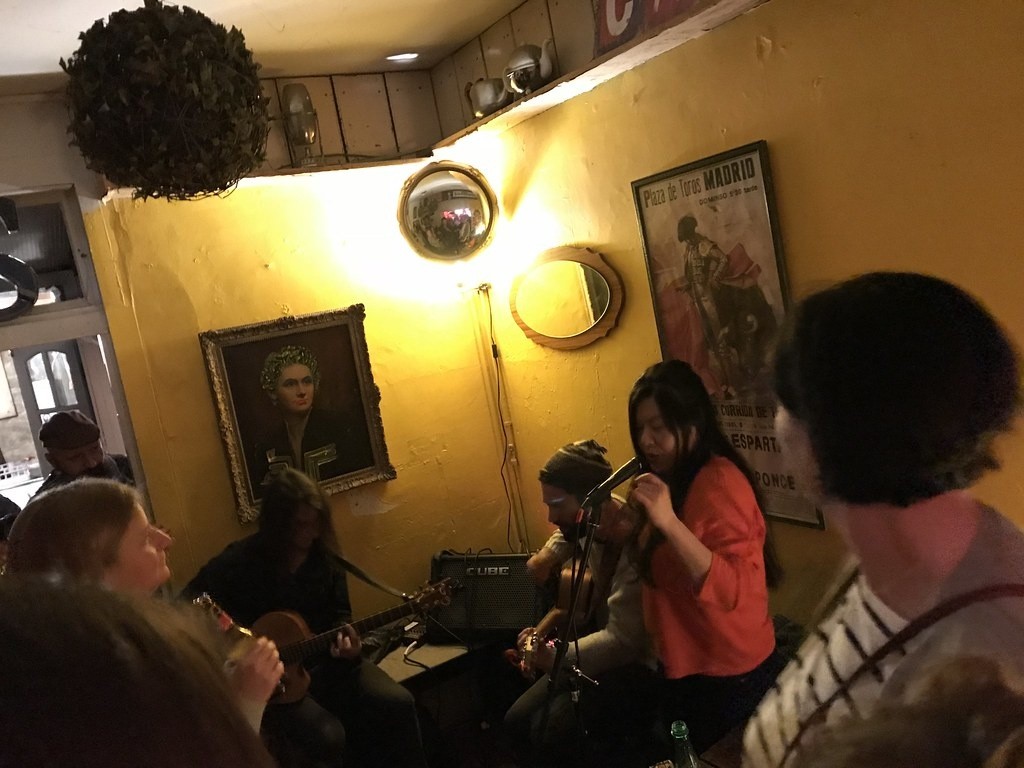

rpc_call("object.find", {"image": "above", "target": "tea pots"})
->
[502,37,553,96]
[464,77,514,120]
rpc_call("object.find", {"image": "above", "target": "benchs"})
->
[379,640,466,698]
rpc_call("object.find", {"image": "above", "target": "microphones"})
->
[580,455,647,511]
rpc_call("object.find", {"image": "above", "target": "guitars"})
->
[249,577,461,705]
[519,558,592,679]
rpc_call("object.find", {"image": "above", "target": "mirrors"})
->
[397,162,499,266]
[509,246,625,350]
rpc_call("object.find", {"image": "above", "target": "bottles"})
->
[671,721,702,768]
[191,592,286,699]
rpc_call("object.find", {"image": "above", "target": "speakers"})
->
[426,552,560,644]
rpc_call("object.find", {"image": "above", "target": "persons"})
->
[0,475,284,768]
[503,441,652,768]
[27,412,137,504]
[180,467,429,768]
[625,359,785,768]
[740,272,1023,768]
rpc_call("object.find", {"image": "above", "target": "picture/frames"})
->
[196,304,397,527]
[630,137,826,532]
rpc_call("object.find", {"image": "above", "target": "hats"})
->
[39,410,100,450]
[539,440,612,498]
